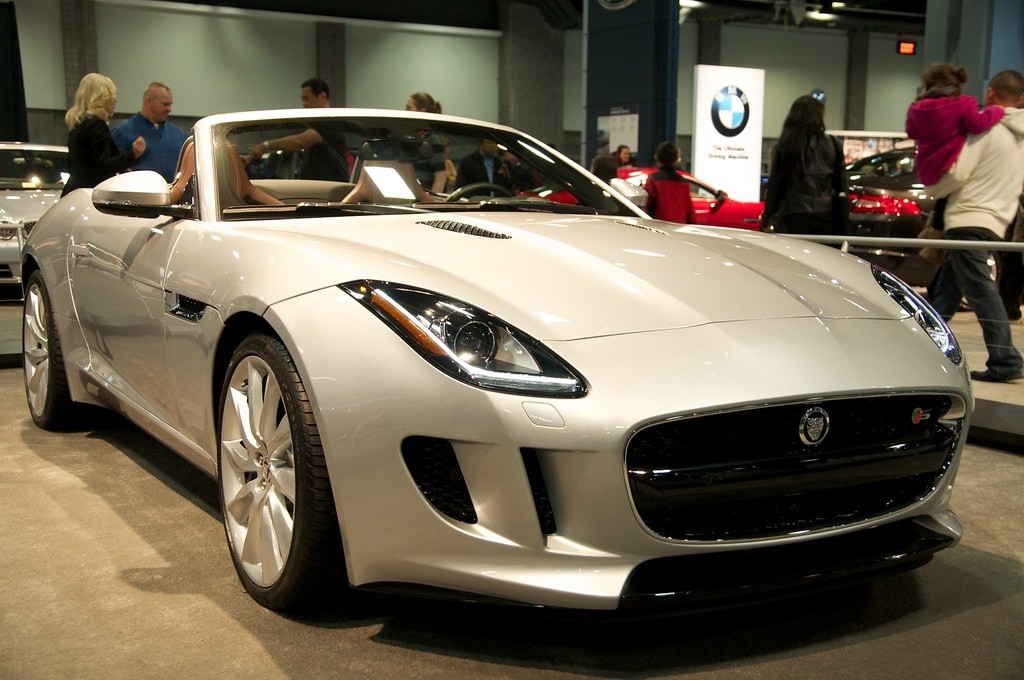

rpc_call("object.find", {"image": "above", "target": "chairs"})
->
[168,134,285,209]
[340,139,437,205]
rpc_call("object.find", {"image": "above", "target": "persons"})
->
[589,153,618,186]
[643,141,699,224]
[60,72,146,200]
[110,82,249,204]
[904,65,1024,382]
[760,96,850,251]
[612,145,637,169]
[248,77,349,184]
[405,92,547,199]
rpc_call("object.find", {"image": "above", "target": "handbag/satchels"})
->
[829,135,850,249]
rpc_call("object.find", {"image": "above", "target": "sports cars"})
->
[20,102,977,624]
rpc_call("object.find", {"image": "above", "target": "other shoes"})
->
[970,367,1024,382]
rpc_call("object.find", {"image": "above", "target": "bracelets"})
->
[261,140,270,149]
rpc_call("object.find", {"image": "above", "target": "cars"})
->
[0,140,71,303]
[842,145,1002,314]
[515,163,765,233]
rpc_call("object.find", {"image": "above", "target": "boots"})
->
[919,227,948,268]
[917,210,935,238]
